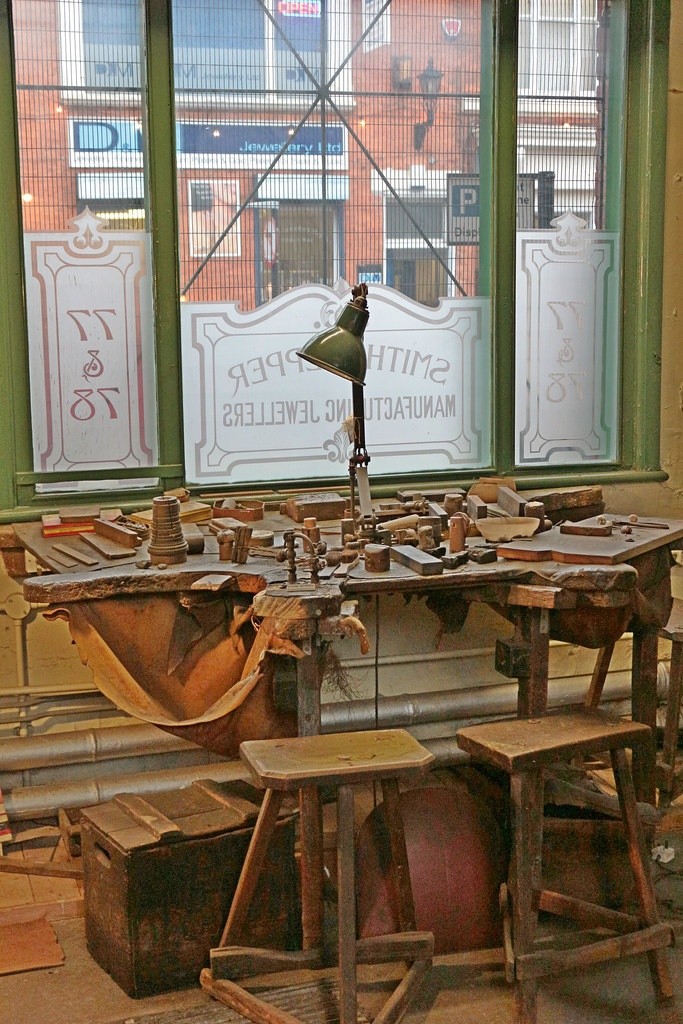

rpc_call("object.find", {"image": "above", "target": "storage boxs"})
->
[78,779,302,1000]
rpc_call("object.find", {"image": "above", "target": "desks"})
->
[0,516,683,947]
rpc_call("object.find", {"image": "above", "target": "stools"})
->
[198,729,436,1024]
[455,707,675,1024]
[585,595,683,810]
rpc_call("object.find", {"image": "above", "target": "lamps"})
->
[414,58,443,151]
[391,55,413,87]
[295,282,383,539]
